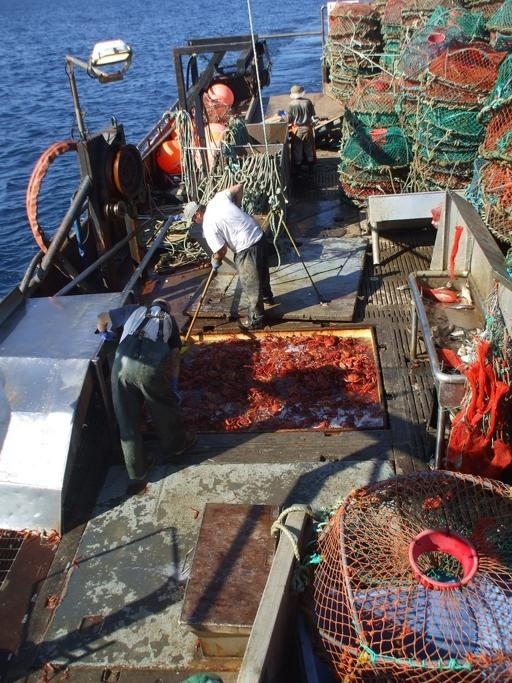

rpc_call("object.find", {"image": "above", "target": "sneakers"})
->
[262,295,274,306]
[126,453,155,490]
[236,314,265,331]
[174,427,197,456]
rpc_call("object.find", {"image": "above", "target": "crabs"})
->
[177,333,379,433]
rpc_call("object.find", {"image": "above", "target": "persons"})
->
[182,183,276,329]
[286,84,317,179]
[96,297,198,494]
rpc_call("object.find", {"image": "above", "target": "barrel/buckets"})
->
[447,10,463,25]
[427,32,446,58]
[369,128,387,143]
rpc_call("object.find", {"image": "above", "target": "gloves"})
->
[211,254,222,273]
[169,380,185,407]
[95,328,118,343]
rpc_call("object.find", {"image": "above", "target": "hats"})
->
[289,85,306,99]
[183,201,202,228]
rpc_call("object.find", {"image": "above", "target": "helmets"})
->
[207,84,235,106]
[150,298,169,312]
